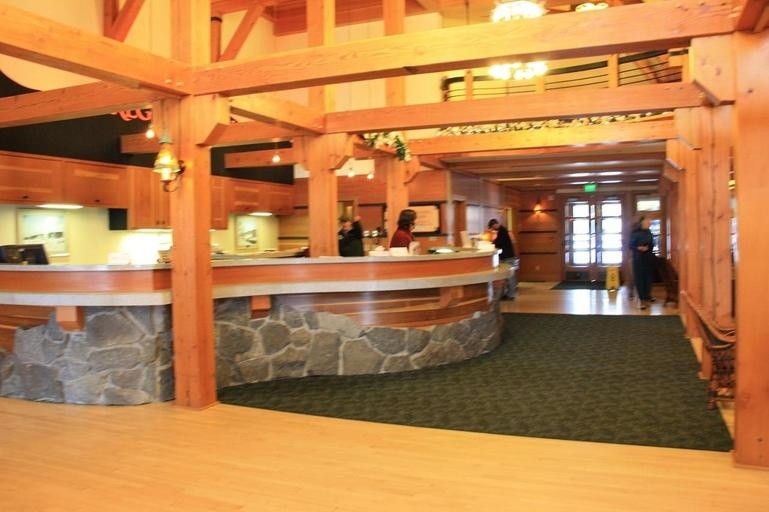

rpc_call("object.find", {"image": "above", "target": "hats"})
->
[338,214,353,222]
[488,219,497,229]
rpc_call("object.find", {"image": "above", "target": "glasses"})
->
[341,220,346,223]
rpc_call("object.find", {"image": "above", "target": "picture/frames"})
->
[16,208,71,259]
[235,215,260,249]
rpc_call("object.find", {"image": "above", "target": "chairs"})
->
[657,256,679,309]
[677,287,739,412]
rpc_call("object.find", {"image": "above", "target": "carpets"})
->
[219,311,734,454]
[551,280,620,290]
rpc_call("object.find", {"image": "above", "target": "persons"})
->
[628,266,657,302]
[628,213,655,310]
[488,218,518,301]
[336,213,365,257]
[390,209,417,250]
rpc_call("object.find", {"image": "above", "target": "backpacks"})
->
[644,254,656,264]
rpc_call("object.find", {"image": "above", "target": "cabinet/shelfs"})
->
[228,177,264,212]
[108,165,171,230]
[64,157,128,210]
[1,152,63,205]
[210,175,227,230]
[264,181,294,216]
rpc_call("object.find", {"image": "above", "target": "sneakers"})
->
[647,296,656,302]
[501,296,514,301]
[640,300,647,310]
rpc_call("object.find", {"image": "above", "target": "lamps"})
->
[152,128,187,192]
